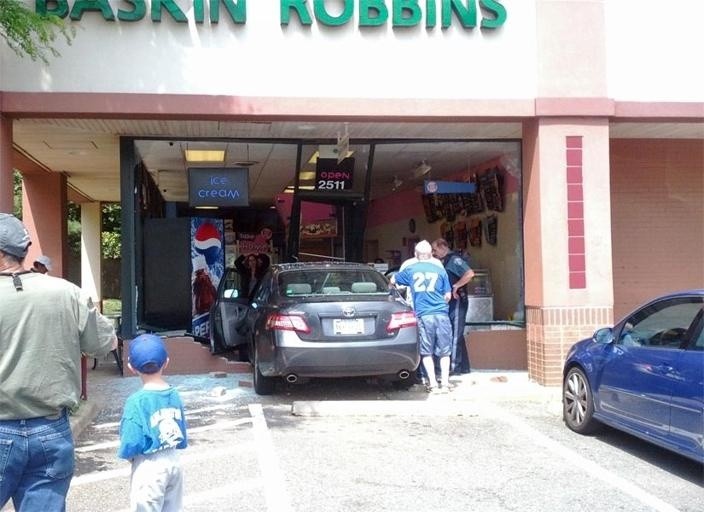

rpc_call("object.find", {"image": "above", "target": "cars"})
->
[563,290,704,464]
[209,261,420,395]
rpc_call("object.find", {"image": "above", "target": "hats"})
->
[127,333,167,374]
[36,256,53,272]
[0,211,32,258]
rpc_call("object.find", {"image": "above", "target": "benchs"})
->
[286,282,380,296]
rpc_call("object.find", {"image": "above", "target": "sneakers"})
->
[450,365,470,375]
[426,382,454,393]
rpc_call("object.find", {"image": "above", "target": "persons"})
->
[396,246,444,310]
[28,255,51,275]
[389,240,453,393]
[116,332,188,512]
[234,254,270,303]
[430,237,474,383]
[0,210,115,511]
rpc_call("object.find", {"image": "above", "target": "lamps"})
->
[414,163,432,177]
[393,177,404,187]
